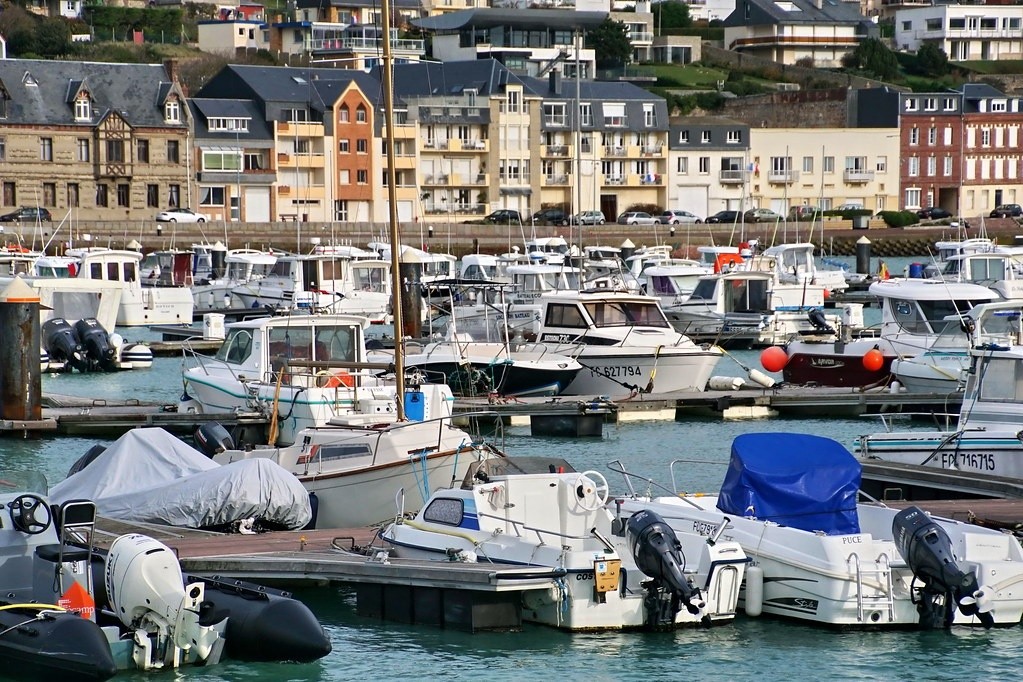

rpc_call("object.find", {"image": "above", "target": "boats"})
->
[598,459,1022,638]
[0,491,334,682]
[0,189,1023,539]
[376,434,754,635]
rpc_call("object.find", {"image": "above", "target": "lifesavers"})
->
[714,253,744,287]
[326,371,354,387]
[739,242,749,254]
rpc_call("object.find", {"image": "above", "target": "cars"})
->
[917,206,954,221]
[0,206,53,223]
[155,208,208,224]
[485,209,522,225]
[705,210,744,224]
[533,207,571,227]
[990,203,1023,218]
[836,203,865,212]
[575,210,606,226]
[618,211,662,225]
[659,210,704,225]
[744,207,786,223]
[789,204,821,221]
